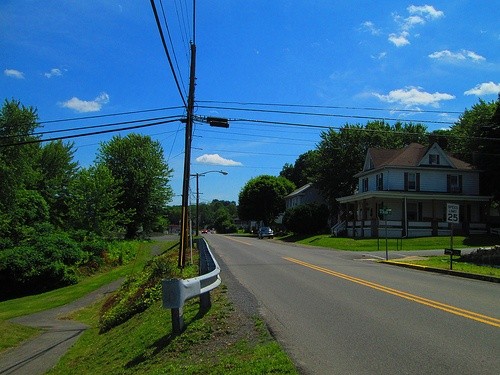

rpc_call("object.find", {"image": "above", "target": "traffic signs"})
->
[446,202,459,223]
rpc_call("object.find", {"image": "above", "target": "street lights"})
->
[189,169,229,236]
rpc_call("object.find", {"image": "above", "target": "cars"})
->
[257,226,274,239]
